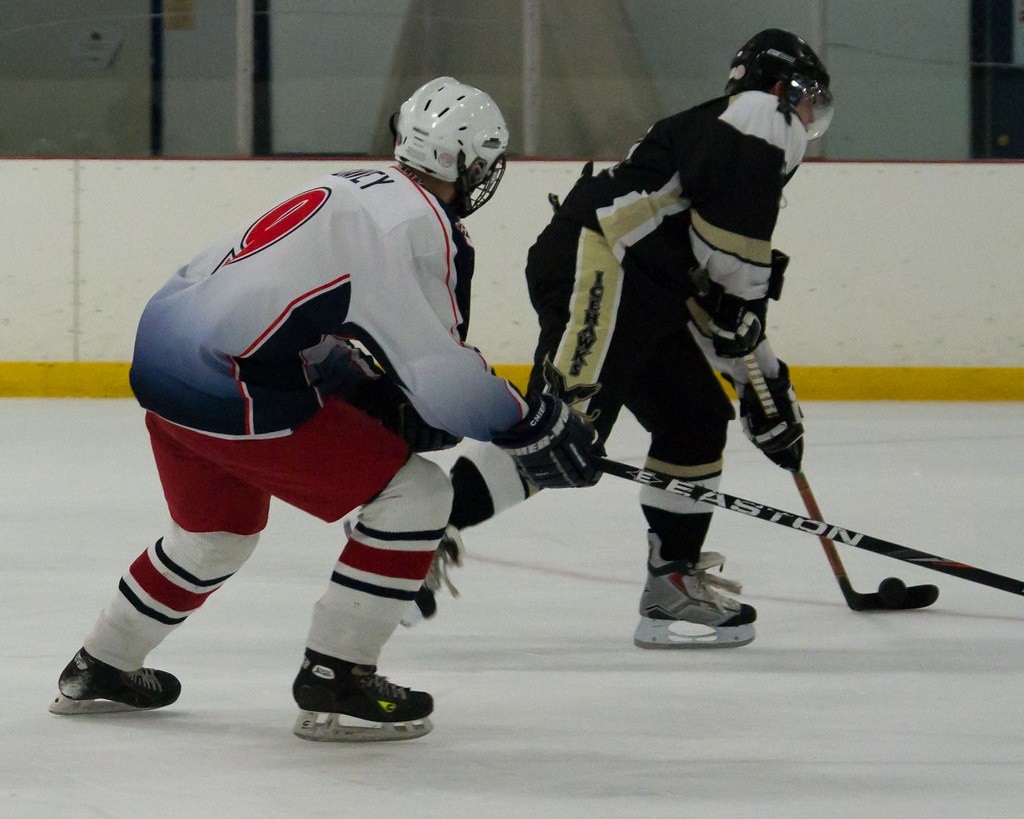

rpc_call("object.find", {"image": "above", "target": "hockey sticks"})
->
[792,470,940,612]
[602,457,1024,594]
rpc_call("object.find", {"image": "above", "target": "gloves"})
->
[709,291,762,359]
[492,392,606,488]
[739,358,806,475]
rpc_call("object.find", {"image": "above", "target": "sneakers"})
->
[345,513,436,630]
[632,528,756,647]
[46,645,182,716]
[292,647,435,742]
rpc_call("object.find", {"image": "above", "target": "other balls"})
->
[877,578,906,591]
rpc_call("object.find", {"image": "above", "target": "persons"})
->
[369,27,829,619]
[53,69,608,724]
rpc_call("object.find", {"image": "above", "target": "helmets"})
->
[724,28,835,140]
[394,74,511,214]
